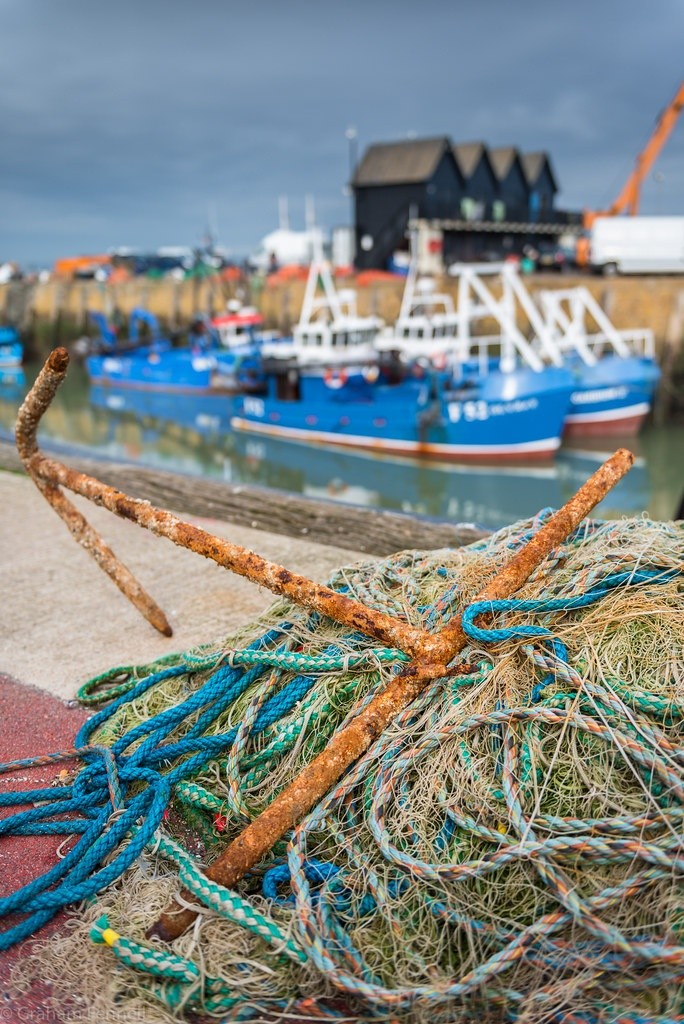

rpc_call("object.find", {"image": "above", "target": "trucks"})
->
[589,217,683,279]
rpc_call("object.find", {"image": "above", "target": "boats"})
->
[79,296,292,397]
[260,227,383,377]
[228,262,574,468]
[437,284,660,445]
[1,324,24,367]
